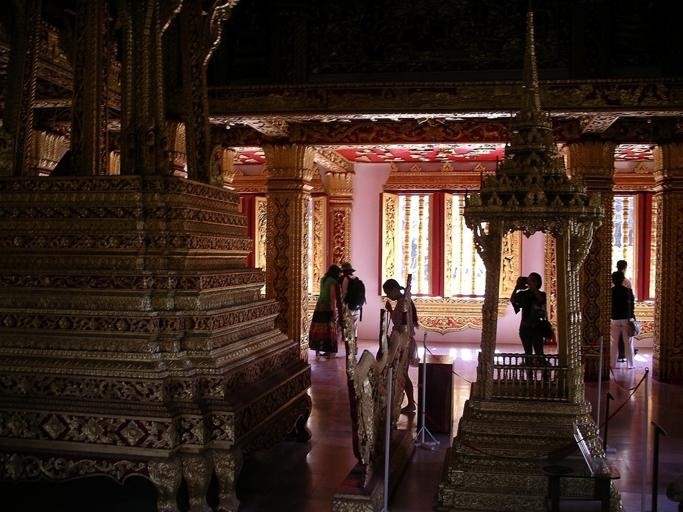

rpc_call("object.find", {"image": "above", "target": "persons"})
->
[410,238,416,270]
[616,259,632,363]
[308,264,341,362]
[609,271,637,368]
[509,272,553,382]
[381,278,419,413]
[338,261,366,358]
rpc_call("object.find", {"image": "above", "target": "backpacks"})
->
[343,275,367,310]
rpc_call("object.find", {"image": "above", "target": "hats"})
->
[339,262,356,272]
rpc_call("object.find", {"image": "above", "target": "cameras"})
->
[520,277,528,284]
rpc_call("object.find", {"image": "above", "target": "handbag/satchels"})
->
[540,320,553,339]
[627,314,640,337]
